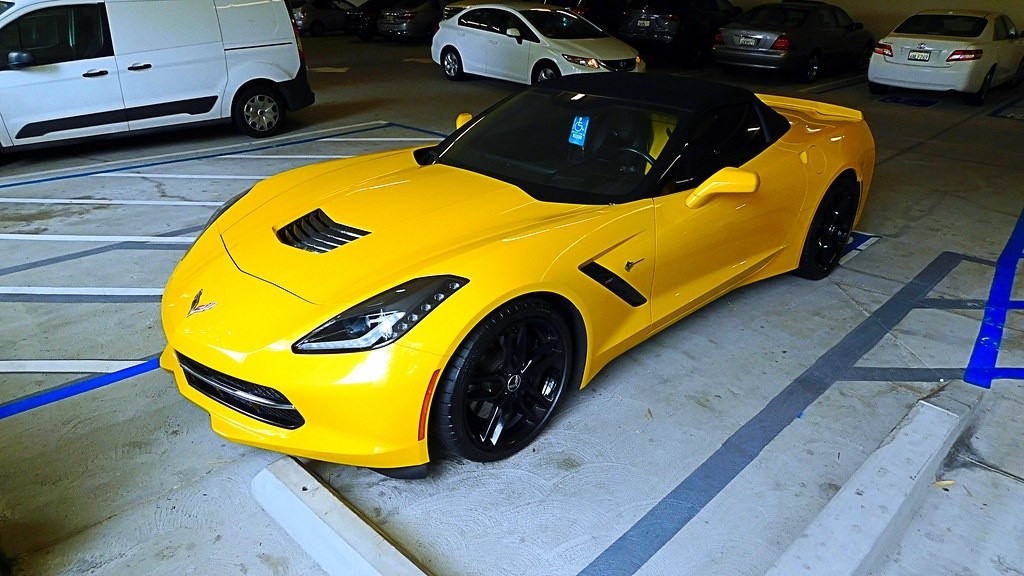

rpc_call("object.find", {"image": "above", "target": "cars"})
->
[441,0,590,22]
[615,0,744,56]
[713,2,876,82]
[869,8,1023,103]
[287,1,352,37]
[376,0,447,40]
[343,0,392,40]
[431,2,645,87]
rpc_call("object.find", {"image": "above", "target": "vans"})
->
[0,2,317,157]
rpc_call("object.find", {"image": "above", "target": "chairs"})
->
[971,20,985,36]
[560,104,651,174]
[922,17,946,35]
[78,15,96,58]
[768,9,788,24]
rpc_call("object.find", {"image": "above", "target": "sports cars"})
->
[152,72,880,479]
